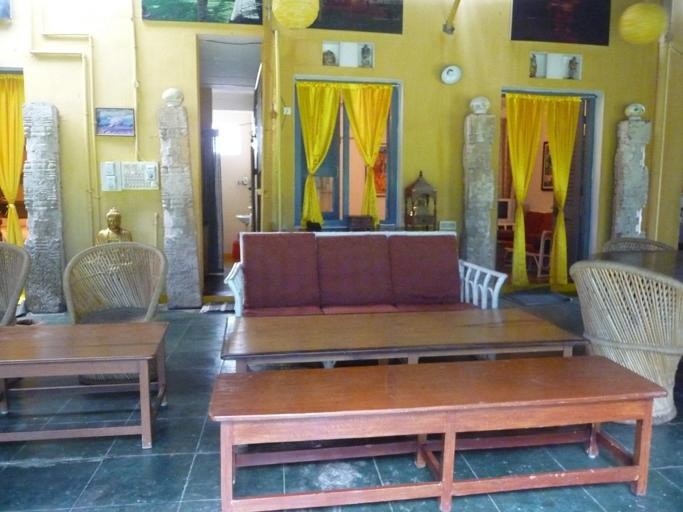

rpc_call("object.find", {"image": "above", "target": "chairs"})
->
[569,237,683,428]
[0,242,170,400]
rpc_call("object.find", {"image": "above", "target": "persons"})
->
[85,207,133,274]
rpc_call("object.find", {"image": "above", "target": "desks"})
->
[0,321,169,449]
[206,354,668,511]
[220,308,591,374]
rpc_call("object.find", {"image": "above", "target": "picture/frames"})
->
[95,107,135,137]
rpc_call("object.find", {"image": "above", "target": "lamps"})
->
[440,64,461,85]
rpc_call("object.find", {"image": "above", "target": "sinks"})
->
[235,213,251,225]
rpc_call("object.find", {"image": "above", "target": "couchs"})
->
[504,210,554,279]
[223,229,509,317]
